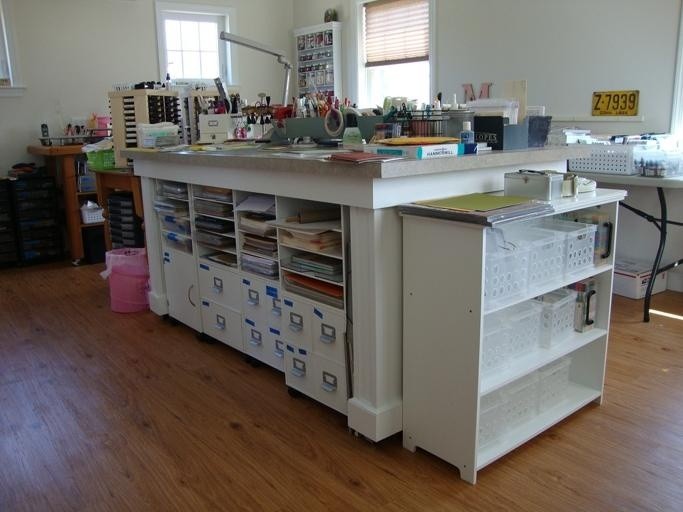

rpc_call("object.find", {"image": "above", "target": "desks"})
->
[87,167,147,251]
[27,145,104,265]
[568,172,683,322]
[118,145,593,447]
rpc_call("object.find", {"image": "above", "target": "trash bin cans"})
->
[100,247,151,315]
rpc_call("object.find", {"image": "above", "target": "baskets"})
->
[138,125,179,147]
[87,149,115,171]
[385,111,444,137]
[568,145,651,175]
[78,176,97,192]
[478,219,597,451]
[79,205,106,224]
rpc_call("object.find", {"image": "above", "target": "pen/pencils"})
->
[291,91,351,119]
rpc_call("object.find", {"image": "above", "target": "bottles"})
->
[297,30,334,99]
[165,72,172,92]
[459,121,475,144]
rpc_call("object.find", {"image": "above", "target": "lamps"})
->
[220,31,292,107]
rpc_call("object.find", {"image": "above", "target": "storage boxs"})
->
[613,258,667,300]
[505,169,563,200]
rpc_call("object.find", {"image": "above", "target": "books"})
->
[151,182,344,309]
[324,136,465,166]
[398,193,554,227]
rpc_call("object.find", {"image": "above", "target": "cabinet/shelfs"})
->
[12,174,60,266]
[0,180,23,269]
[292,21,342,107]
[396,188,628,486]
[109,86,240,170]
